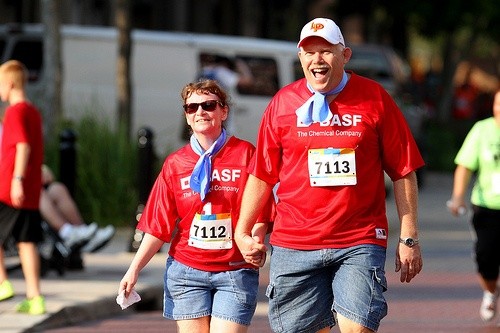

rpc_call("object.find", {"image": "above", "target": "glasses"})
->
[182,100,223,114]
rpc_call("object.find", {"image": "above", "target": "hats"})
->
[297,18,345,48]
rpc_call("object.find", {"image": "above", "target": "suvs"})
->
[0,20,56,120]
[342,46,432,187]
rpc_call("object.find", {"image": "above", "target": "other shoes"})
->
[479,286,500,322]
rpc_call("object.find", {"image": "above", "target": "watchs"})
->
[399,236,419,247]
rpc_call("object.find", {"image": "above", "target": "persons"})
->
[444,92,499,324]
[55,128,86,215]
[392,44,438,191]
[235,17,426,333]
[449,42,498,163]
[200,48,250,110]
[36,163,116,253]
[0,60,48,317]
[124,123,165,255]
[117,75,276,333]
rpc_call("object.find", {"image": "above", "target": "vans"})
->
[55,26,397,195]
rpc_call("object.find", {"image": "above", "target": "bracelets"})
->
[12,175,24,182]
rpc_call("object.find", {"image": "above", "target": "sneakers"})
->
[63,221,116,254]
[1,282,47,316]
